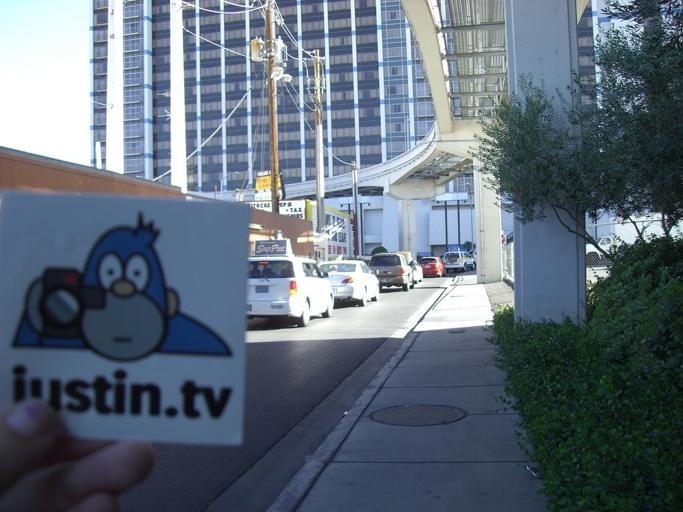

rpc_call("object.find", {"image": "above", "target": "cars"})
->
[245,251,476,327]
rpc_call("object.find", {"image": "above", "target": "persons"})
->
[0,398,153,512]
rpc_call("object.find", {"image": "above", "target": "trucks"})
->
[585,213,683,268]
